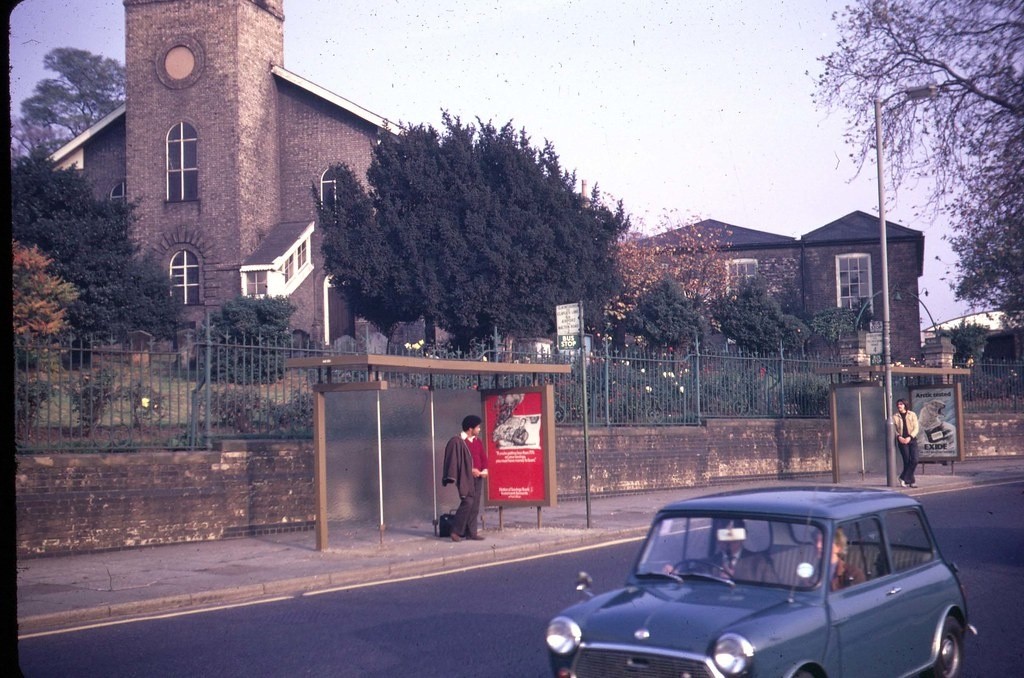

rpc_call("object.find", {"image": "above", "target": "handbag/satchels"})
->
[439,509,469,538]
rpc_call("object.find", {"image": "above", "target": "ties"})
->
[726,557,736,571]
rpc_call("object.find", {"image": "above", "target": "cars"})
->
[544,486,979,678]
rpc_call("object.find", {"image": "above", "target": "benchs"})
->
[767,544,929,590]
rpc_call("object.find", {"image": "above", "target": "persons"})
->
[893,398,919,487]
[442,415,488,541]
[803,525,865,592]
[663,518,780,583]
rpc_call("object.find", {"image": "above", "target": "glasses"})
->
[897,403,902,406]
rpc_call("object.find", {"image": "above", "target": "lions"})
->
[918,401,956,449]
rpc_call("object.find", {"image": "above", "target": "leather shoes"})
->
[450,531,463,542]
[466,535,485,540]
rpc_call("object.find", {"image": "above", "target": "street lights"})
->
[873,84,939,486]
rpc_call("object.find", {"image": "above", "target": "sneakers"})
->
[898,476,906,487]
[909,483,918,487]
